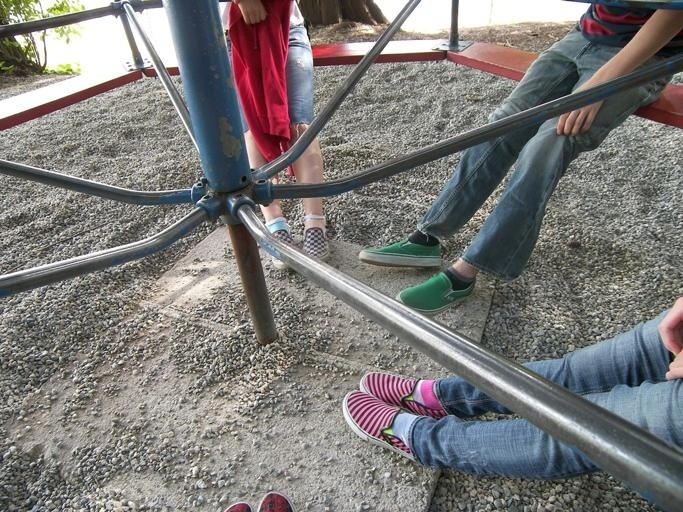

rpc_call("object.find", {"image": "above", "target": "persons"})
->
[217,1,332,272]
[220,491,299,512]
[356,1,683,318]
[338,292,683,481]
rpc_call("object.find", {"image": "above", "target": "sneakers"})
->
[359,238,441,267]
[302,226,330,260]
[223,491,295,512]
[360,371,447,420]
[396,272,476,315]
[342,389,421,464]
[271,224,296,270]
